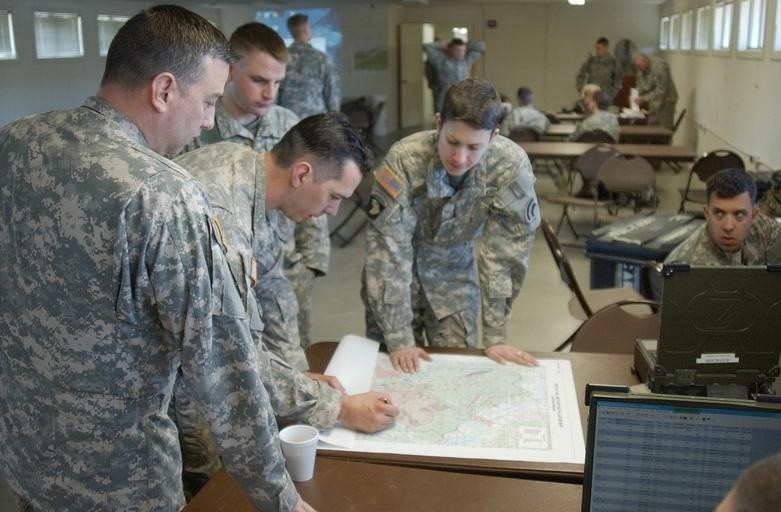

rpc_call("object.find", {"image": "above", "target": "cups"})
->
[277,424,320,482]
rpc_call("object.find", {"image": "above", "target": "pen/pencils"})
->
[384,398,388,404]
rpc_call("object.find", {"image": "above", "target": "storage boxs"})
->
[584,209,707,293]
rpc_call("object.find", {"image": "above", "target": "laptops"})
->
[632,262,780,391]
[581,390,780,511]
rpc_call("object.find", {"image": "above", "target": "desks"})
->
[182,341,781,512]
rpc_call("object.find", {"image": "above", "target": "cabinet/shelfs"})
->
[400,23,436,129]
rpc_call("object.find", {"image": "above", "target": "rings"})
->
[519,351,522,356]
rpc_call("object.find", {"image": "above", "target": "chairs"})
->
[346,97,384,156]
[329,185,373,247]
[503,98,745,249]
[541,219,654,352]
[570,299,660,355]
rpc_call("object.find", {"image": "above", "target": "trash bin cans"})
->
[371,95,389,135]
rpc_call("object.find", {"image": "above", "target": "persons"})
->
[631,51,680,146]
[423,36,487,114]
[576,36,625,111]
[362,78,543,374]
[272,12,343,124]
[0,4,315,512]
[174,22,333,354]
[572,84,603,115]
[158,110,400,501]
[499,85,552,145]
[712,447,781,511]
[665,167,781,267]
[562,88,622,196]
[425,35,444,117]
